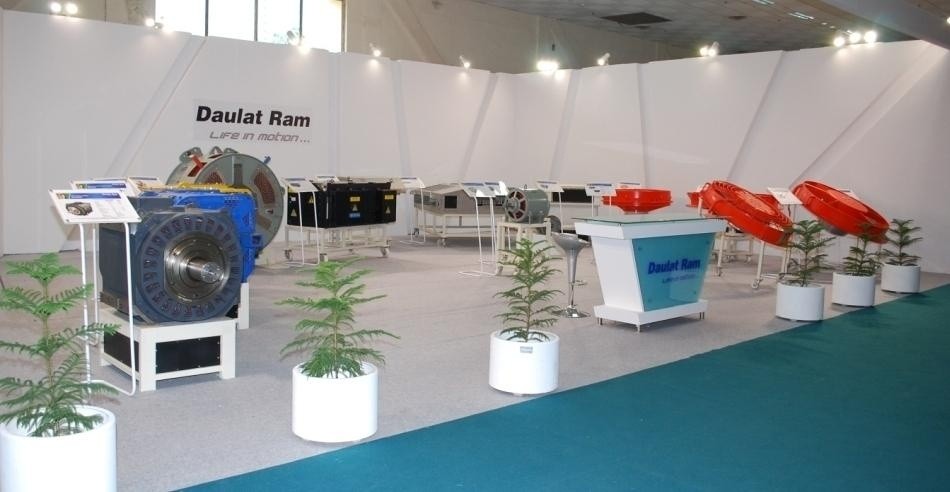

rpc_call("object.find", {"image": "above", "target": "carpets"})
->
[168,283,950,492]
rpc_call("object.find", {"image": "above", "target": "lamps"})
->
[700,41,720,57]
[367,43,381,57]
[833,30,850,49]
[598,53,611,66]
[458,55,471,68]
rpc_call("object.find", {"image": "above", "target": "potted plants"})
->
[1,252,124,492]
[775,217,923,323]
[275,255,400,444]
[488,234,566,395]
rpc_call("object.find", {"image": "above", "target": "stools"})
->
[549,231,591,318]
